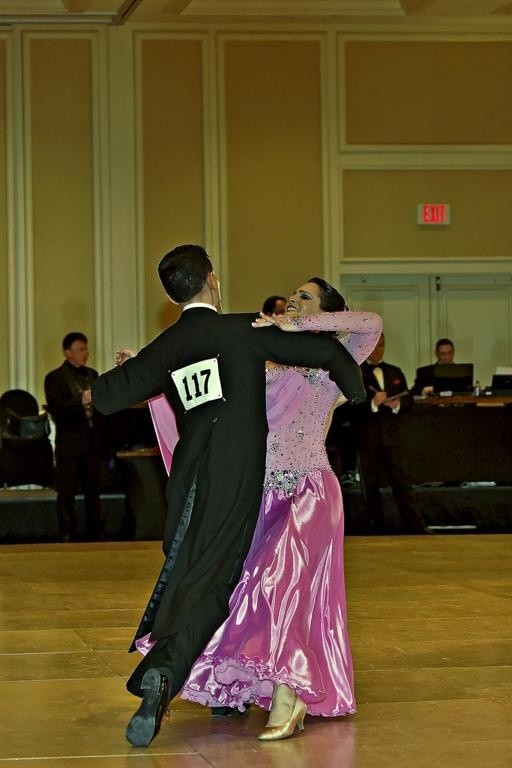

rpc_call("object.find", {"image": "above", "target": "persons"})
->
[43,331,108,542]
[115,277,383,742]
[348,333,432,535]
[410,337,454,396]
[262,294,289,316]
[89,244,368,748]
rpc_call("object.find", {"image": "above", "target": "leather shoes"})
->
[123,665,169,749]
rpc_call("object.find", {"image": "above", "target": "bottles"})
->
[473,381,483,399]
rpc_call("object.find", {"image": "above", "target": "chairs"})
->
[3,387,54,486]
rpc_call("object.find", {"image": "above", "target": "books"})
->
[381,391,409,405]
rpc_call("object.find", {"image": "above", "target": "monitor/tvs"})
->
[427,363,475,396]
[483,374,512,396]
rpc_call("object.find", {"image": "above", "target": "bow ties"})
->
[369,362,385,370]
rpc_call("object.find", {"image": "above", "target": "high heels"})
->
[257,693,307,740]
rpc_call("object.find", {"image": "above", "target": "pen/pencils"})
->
[369,386,377,393]
